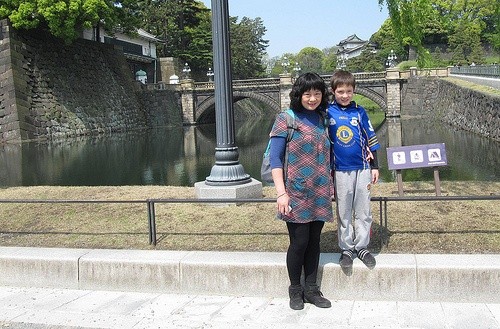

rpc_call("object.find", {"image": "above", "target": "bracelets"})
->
[276,192,286,199]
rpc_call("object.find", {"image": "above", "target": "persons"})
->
[455,61,476,75]
[268,72,374,310]
[327,70,381,267]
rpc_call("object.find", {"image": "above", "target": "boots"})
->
[305,286,331,308]
[289,285,304,310]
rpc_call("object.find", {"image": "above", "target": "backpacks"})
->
[261,109,329,186]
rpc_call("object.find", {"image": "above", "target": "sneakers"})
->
[357,248,376,265]
[339,250,354,267]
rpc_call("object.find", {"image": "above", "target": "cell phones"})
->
[284,204,293,215]
